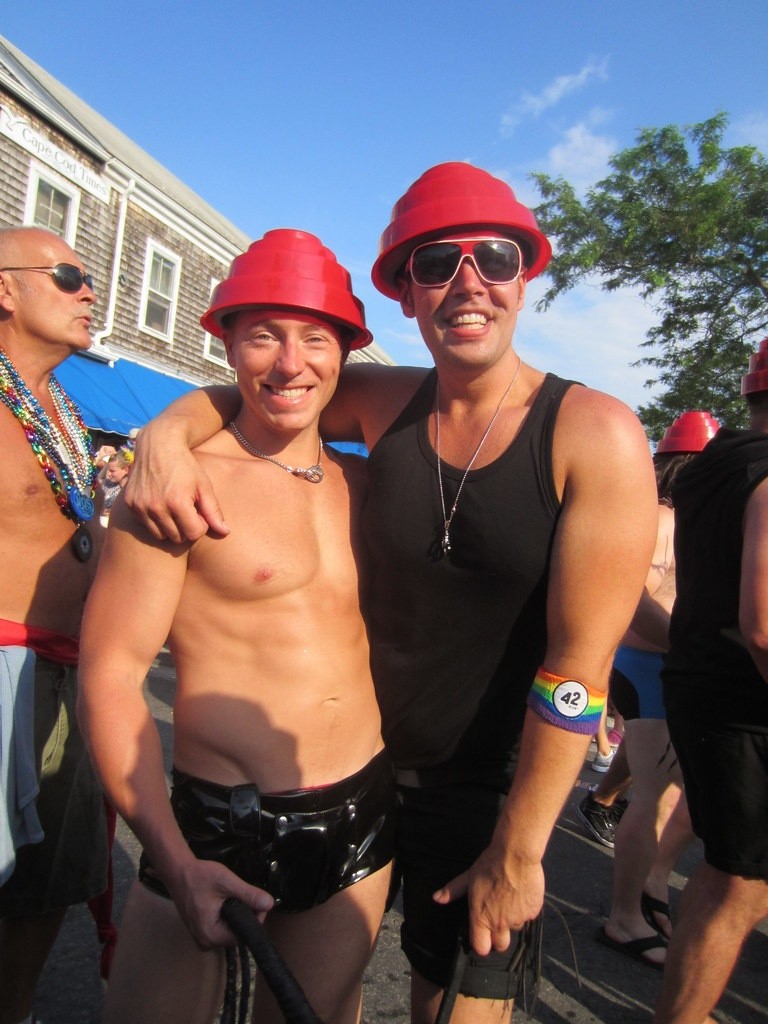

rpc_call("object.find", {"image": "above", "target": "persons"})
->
[575,346,768,1024]
[91,428,140,518]
[126,162,658,1024]
[74,229,406,1023]
[0,227,107,1024]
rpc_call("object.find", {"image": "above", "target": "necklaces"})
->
[229,421,323,483]
[0,350,97,562]
[436,356,520,553]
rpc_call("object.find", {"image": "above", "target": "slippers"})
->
[595,926,665,972]
[642,892,673,941]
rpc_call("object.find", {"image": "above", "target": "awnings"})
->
[53,353,199,438]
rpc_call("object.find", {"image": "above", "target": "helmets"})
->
[652,411,721,455]
[369,161,553,302]
[740,334,768,396]
[199,227,375,352]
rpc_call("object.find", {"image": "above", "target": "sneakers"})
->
[575,790,628,849]
[608,728,624,747]
[591,748,618,773]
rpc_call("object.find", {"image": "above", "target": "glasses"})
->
[403,236,528,289]
[227,782,269,884]
[0,262,93,293]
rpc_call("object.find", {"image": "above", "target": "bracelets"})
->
[527,668,607,736]
